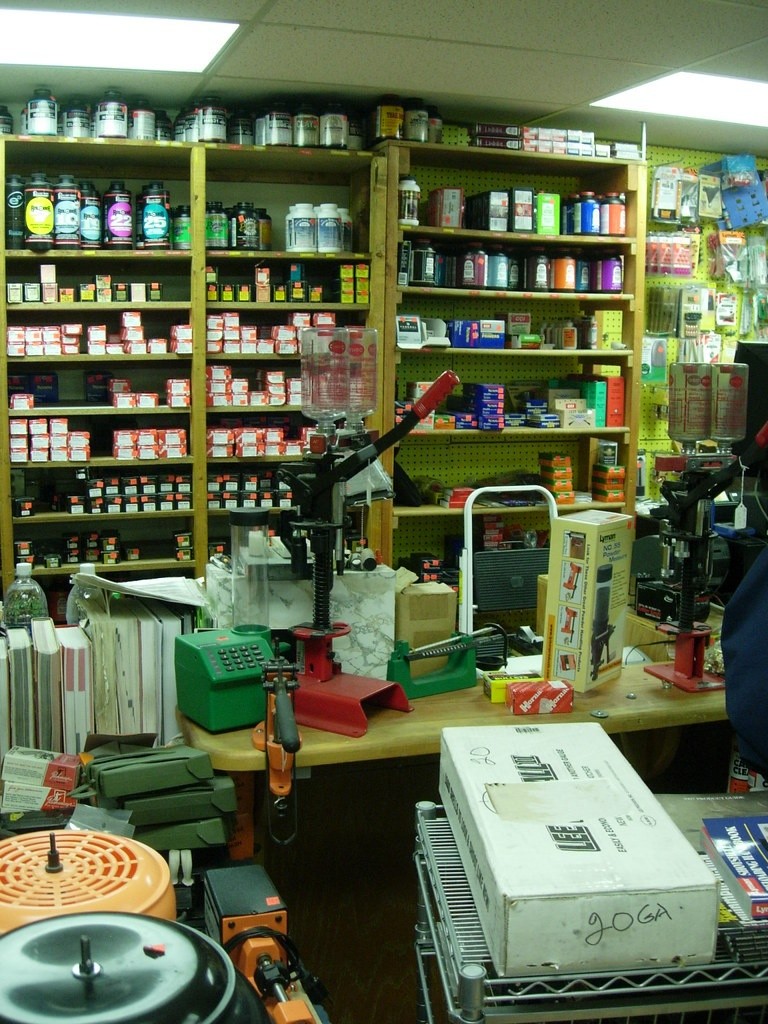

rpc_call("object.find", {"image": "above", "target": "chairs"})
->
[457,486,557,634]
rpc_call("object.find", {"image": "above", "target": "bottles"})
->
[524,246,589,294]
[104,182,132,250]
[372,94,442,146]
[563,192,627,237]
[398,177,420,226]
[174,97,254,146]
[410,238,518,291]
[66,563,102,625]
[174,202,272,252]
[590,247,622,294]
[0,106,12,133]
[4,562,48,637]
[20,89,172,141]
[256,101,364,150]
[6,173,54,249]
[54,175,102,250]
[136,182,170,250]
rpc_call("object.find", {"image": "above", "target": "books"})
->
[699,815,768,920]
[0,597,195,756]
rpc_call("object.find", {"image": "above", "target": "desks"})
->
[175,573,728,770]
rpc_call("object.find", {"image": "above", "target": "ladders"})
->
[459,485,558,637]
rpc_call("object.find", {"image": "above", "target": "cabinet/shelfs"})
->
[413,801,768,1024]
[0,134,387,607]
[363,139,651,605]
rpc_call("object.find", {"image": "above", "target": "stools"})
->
[476,646,655,781]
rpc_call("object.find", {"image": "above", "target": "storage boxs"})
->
[468,123,639,160]
[394,374,625,429]
[539,453,625,504]
[1,746,81,811]
[14,530,140,569]
[636,581,711,623]
[10,417,187,463]
[6,264,163,304]
[482,510,634,715]
[7,312,193,357]
[8,371,58,410]
[439,723,720,977]
[394,313,541,350]
[206,414,380,458]
[205,365,362,407]
[83,371,191,408]
[410,515,549,611]
[172,530,194,561]
[425,489,474,508]
[207,313,365,354]
[208,526,276,561]
[428,187,560,236]
[395,580,457,678]
[207,469,292,509]
[206,264,370,303]
[11,467,192,518]
[345,533,368,552]
